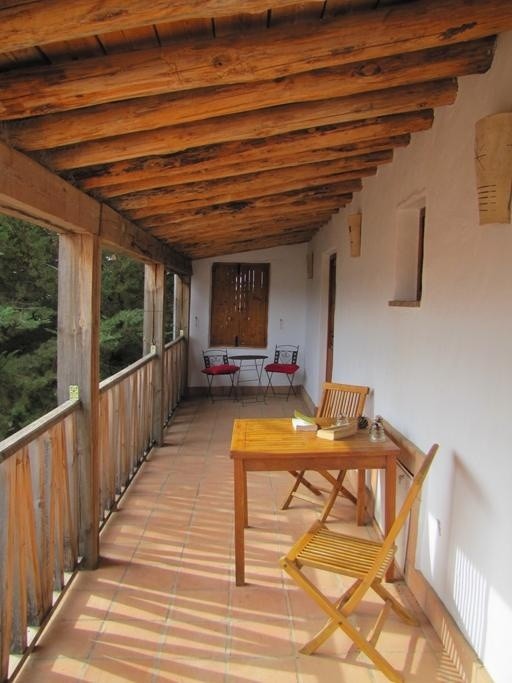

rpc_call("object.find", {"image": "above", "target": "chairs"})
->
[282,382,369,525]
[263,344,299,402]
[279,443,439,683]
[200,346,240,403]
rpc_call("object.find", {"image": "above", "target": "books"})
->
[291,409,359,441]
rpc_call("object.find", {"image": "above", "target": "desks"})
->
[229,355,270,406]
[230,418,400,586]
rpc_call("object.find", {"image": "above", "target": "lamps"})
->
[306,251,314,279]
[347,214,361,256]
[475,111,512,225]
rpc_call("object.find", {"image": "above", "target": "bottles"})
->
[336,413,349,425]
[371,415,385,443]
